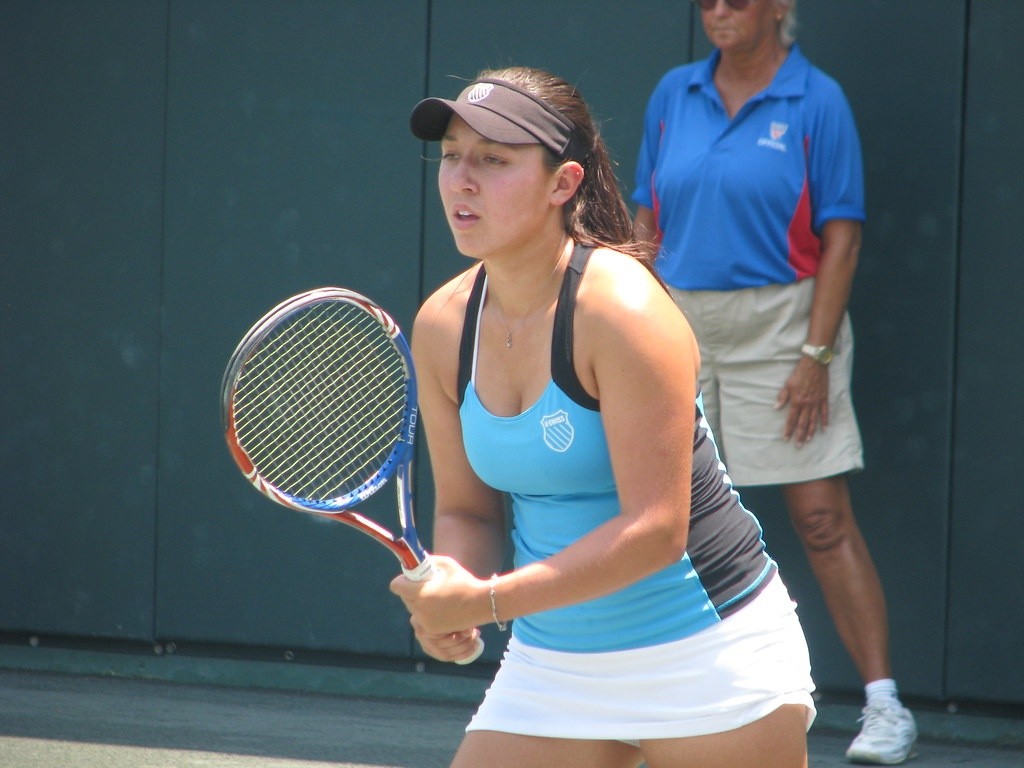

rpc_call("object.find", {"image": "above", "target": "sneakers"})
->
[844,702,918,765]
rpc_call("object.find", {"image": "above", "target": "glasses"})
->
[699,0,751,12]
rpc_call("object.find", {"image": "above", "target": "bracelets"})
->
[489,572,508,631]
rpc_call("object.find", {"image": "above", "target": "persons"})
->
[389,65,819,768]
[631,1,919,765]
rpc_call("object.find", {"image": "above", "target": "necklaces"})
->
[492,244,567,349]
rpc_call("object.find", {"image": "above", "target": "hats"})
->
[407,79,577,160]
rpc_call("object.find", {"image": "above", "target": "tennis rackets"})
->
[221,283,483,664]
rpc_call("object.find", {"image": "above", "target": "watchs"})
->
[800,343,833,366]
